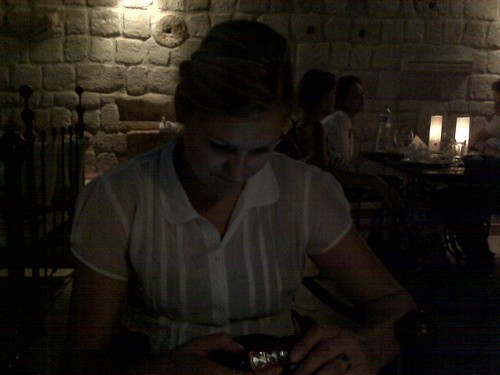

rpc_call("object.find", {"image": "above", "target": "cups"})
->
[450,140,467,160]
[425,142,441,161]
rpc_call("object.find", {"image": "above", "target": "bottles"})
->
[375,112,392,153]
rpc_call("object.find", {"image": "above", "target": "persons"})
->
[452,80,500,269]
[319,74,368,167]
[297,69,399,209]
[68,21,417,375]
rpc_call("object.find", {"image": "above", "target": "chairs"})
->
[26,279,403,375]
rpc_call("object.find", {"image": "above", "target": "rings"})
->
[340,352,352,372]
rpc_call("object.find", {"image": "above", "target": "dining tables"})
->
[362,151,466,246]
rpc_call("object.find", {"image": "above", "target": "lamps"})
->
[454,116,471,152]
[428,114,443,152]
